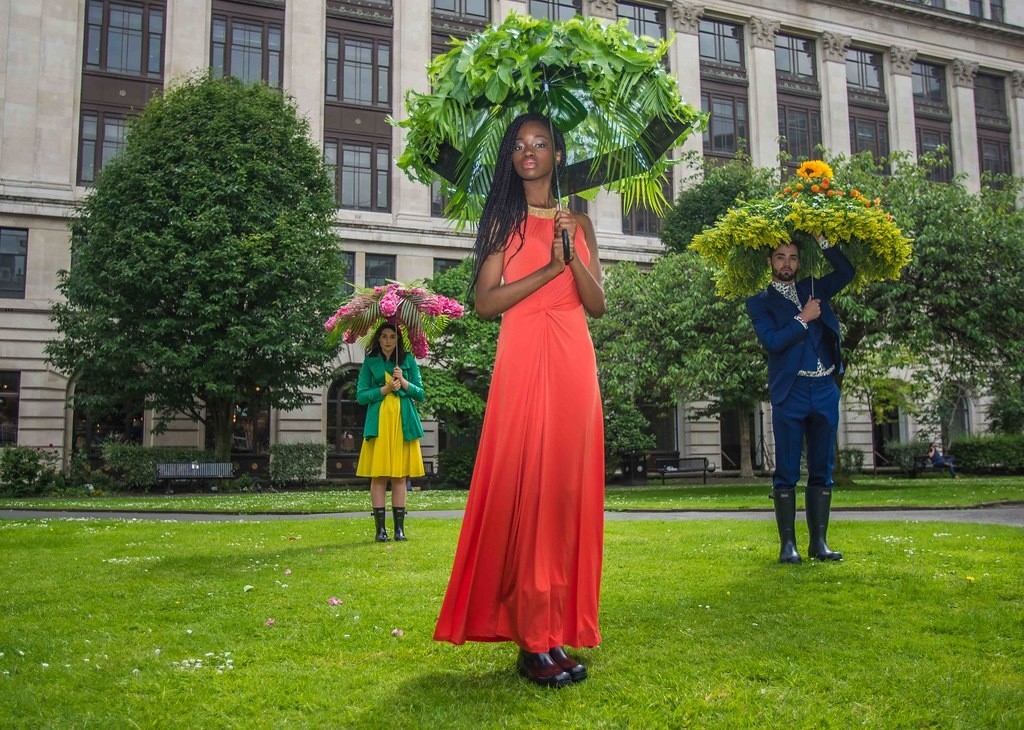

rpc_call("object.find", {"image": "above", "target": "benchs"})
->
[654,456,716,484]
[912,454,959,478]
[413,462,437,490]
[157,462,236,495]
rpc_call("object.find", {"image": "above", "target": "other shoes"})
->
[954,474,959,479]
[944,461,952,467]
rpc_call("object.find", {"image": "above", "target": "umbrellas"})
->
[324,280,465,381]
[383,9,711,266]
[690,159,917,304]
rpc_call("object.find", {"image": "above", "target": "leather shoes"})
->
[551,648,589,681]
[516,648,571,687]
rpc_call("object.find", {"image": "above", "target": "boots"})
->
[806,485,843,560]
[392,507,407,541]
[373,508,391,542]
[772,486,803,562]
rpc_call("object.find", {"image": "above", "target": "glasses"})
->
[933,445,937,448]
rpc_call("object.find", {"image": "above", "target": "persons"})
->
[354,323,427,543]
[0,414,361,455]
[927,442,959,480]
[430,113,608,688]
[746,229,856,562]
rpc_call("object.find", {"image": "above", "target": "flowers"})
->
[324,277,466,358]
[689,160,917,304]
